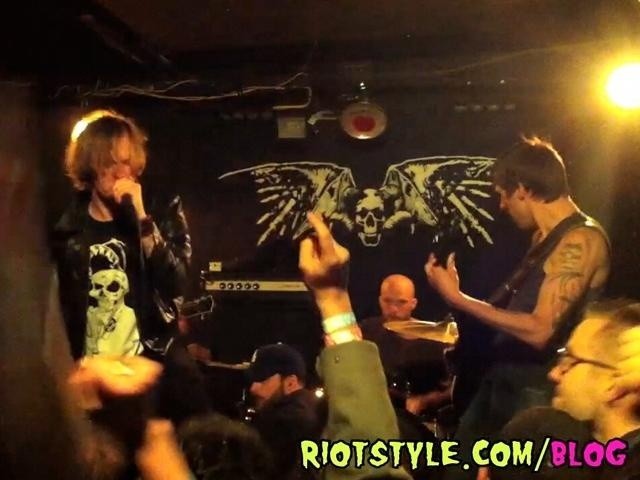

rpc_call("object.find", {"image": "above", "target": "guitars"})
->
[434,234,494,411]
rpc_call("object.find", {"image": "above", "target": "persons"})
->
[45,106,191,362]
[423,128,614,479]
[0,201,640,480]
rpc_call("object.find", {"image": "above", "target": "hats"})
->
[235,343,305,382]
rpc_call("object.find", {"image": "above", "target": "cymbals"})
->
[382,319,460,345]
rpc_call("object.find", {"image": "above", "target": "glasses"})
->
[552,346,616,373]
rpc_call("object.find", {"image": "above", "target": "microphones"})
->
[118,194,137,220]
